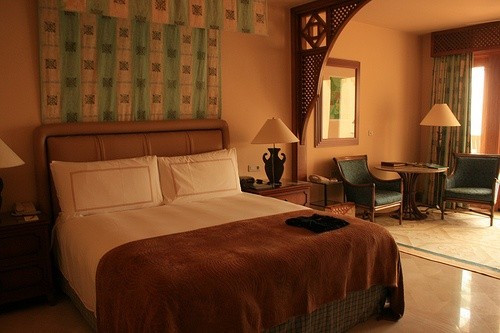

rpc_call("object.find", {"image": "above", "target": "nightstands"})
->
[0,212,56,306]
[245,179,312,208]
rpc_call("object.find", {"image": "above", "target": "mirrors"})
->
[314,58,361,148]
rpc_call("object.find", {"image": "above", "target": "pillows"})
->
[51,154,163,219]
[158,147,241,204]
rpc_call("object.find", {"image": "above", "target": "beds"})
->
[33,120,404,333]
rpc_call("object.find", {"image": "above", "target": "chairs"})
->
[442,152,500,227]
[333,155,403,224]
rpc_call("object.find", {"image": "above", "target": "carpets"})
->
[363,208,500,280]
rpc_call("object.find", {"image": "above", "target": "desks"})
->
[376,164,447,221]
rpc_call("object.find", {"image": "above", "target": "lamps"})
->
[0,139,26,208]
[418,103,462,215]
[251,117,300,185]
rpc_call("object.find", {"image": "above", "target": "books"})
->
[381,161,407,166]
[429,165,450,169]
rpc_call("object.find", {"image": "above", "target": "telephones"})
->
[309,174,331,183]
[13,199,37,214]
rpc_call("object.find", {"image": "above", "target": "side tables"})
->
[309,181,344,207]
